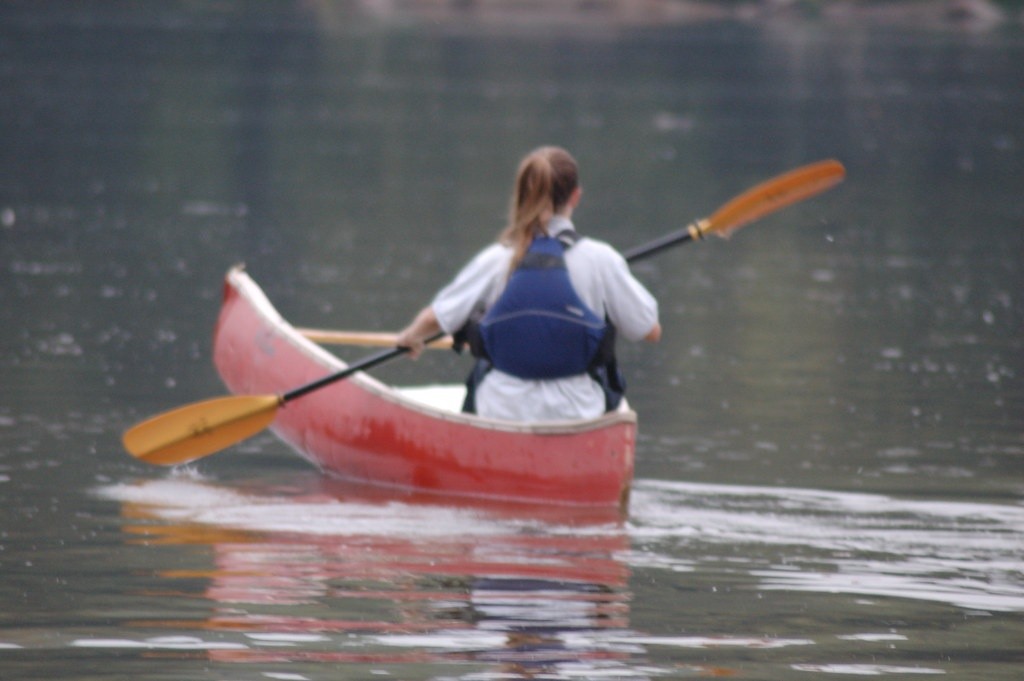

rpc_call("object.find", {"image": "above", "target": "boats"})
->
[209,258,640,505]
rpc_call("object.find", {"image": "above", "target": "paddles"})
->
[122,159,845,468]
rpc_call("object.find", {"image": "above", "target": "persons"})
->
[392,146,663,423]
